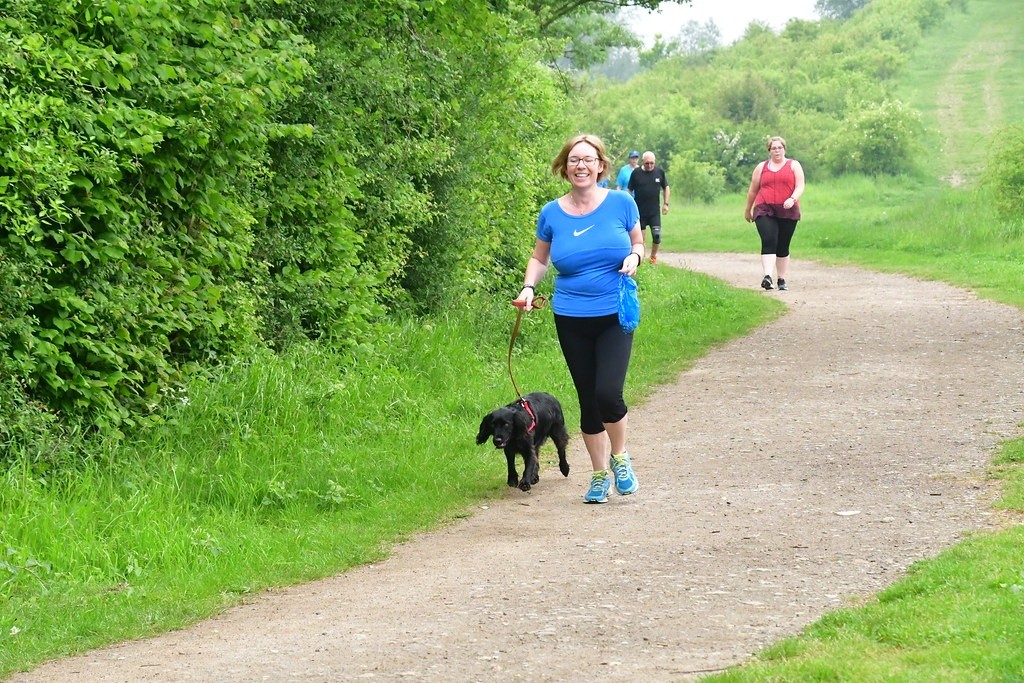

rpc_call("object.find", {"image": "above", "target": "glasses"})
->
[566,154,599,166]
[770,146,784,150]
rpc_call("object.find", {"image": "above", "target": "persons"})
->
[515,134,646,503]
[597,150,640,197]
[745,136,805,290]
[627,151,669,266]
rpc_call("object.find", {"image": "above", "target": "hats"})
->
[629,150,639,158]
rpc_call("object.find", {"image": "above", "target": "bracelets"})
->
[520,284,535,292]
[630,252,641,268]
[664,202,668,206]
[790,196,797,202]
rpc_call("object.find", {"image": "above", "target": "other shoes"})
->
[650,255,656,265]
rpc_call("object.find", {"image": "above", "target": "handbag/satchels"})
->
[617,273,640,334]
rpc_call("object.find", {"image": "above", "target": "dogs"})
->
[476,390,571,492]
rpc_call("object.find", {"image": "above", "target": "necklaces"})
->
[569,192,587,215]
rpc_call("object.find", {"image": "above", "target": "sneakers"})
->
[761,274,774,289]
[583,470,612,503]
[610,449,638,494]
[777,278,788,289]
